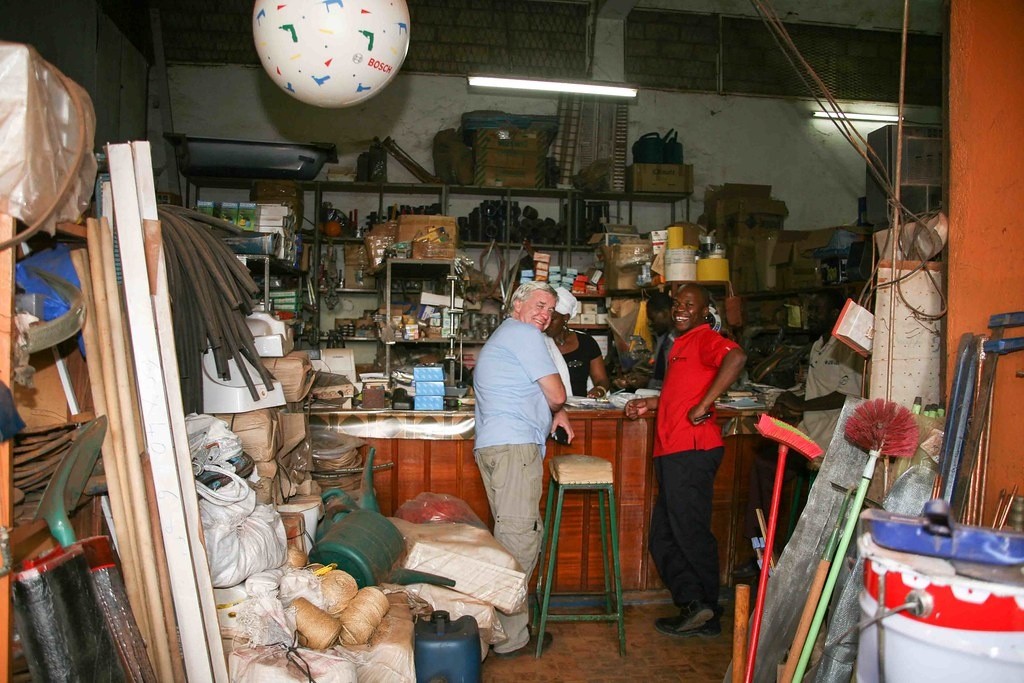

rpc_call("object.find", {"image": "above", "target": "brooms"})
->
[745,413,826,681]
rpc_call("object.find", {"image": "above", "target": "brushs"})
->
[793,399,919,683]
[745,414,823,683]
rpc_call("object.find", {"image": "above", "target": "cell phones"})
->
[552,426,571,445]
[694,412,714,421]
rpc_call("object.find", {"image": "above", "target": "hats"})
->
[551,287,577,320]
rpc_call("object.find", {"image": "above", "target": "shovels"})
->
[860,499,1023,568]
[33,416,106,547]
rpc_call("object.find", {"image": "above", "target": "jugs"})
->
[309,446,458,589]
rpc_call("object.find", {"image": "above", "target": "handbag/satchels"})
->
[186,411,250,505]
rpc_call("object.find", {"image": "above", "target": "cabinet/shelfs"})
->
[715,286,839,372]
[183,176,690,404]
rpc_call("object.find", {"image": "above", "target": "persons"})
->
[613,293,676,391]
[771,291,864,460]
[625,283,747,638]
[544,287,610,398]
[472,281,575,659]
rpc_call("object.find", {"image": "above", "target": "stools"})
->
[529,454,627,657]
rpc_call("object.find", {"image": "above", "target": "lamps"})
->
[811,111,905,123]
[469,74,638,98]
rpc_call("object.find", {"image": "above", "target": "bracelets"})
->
[596,386,606,393]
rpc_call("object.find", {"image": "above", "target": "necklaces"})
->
[556,338,565,345]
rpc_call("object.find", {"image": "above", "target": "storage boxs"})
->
[256,205,289,215]
[272,298,298,304]
[238,202,255,232]
[220,202,238,223]
[196,199,215,216]
[259,227,283,234]
[273,305,304,311]
[269,289,298,297]
[696,184,865,292]
[258,218,284,226]
[333,121,866,409]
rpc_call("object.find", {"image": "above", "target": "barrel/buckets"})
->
[414,609,482,683]
[854,573,1024,683]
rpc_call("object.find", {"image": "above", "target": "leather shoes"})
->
[676,600,714,632]
[489,624,533,649]
[654,616,721,637]
[495,631,552,659]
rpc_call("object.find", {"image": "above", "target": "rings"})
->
[598,392,600,395]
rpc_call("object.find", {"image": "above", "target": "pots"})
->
[861,500,1024,567]
